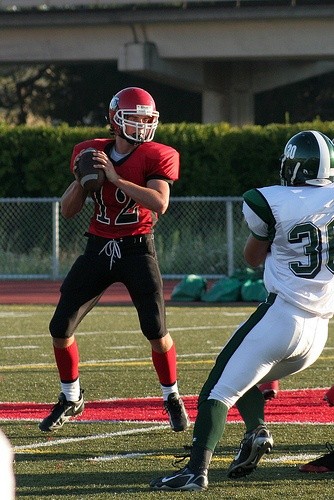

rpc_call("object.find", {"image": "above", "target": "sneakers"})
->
[38,387,85,431]
[227,425,274,480]
[150,466,209,490]
[298,442,334,472]
[161,393,191,431]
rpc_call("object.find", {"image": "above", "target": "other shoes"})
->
[258,381,278,402]
[323,384,334,406]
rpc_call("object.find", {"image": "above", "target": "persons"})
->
[36,88,191,432]
[147,131,334,491]
[299,386,334,472]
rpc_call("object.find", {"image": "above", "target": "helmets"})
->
[280,130,334,186]
[108,87,160,145]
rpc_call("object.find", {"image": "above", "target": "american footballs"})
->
[74,147,105,193]
